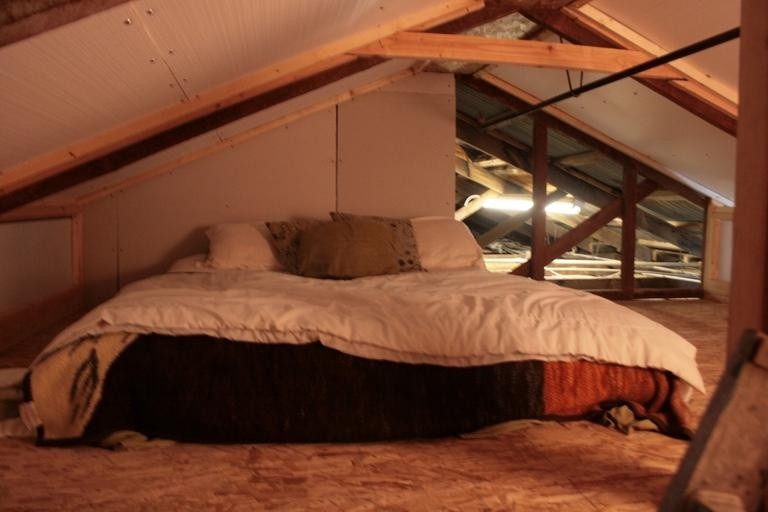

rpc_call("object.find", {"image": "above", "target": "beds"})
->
[26,216,702,445]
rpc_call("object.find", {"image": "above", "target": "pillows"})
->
[195,212,485,280]
